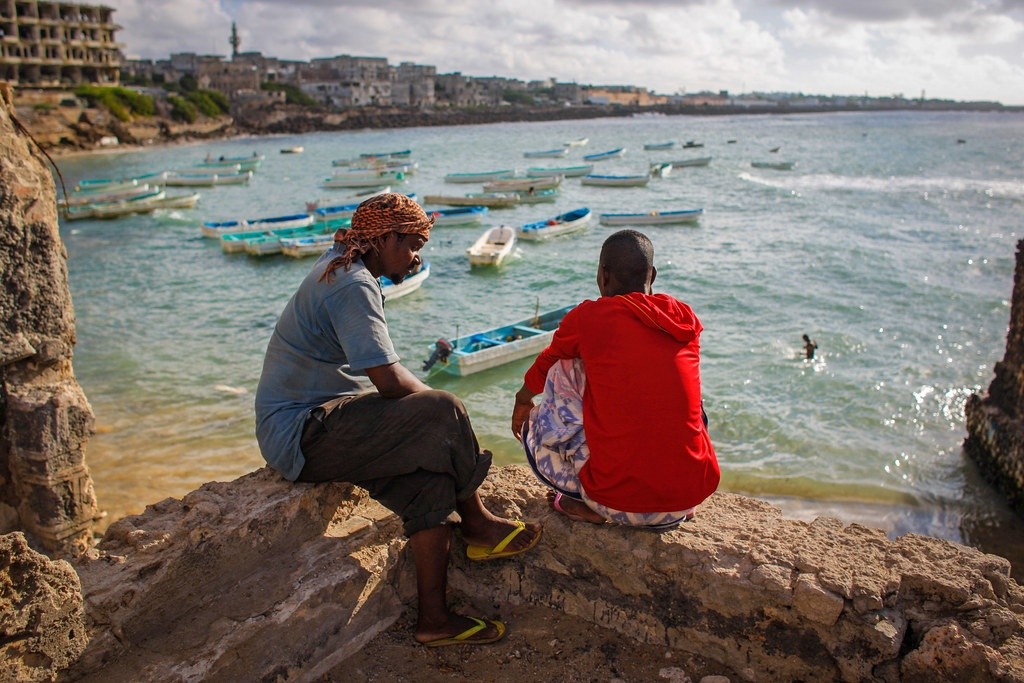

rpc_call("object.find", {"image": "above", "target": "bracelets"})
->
[515,393,531,405]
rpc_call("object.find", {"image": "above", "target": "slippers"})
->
[423,615,505,646]
[552,489,587,523]
[467,520,543,561]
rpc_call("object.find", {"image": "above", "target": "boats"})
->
[598,206,704,227]
[562,137,590,148]
[580,146,627,162]
[420,293,580,376]
[54,143,567,263]
[514,205,594,243]
[522,147,569,158]
[682,140,705,149]
[581,173,651,186]
[463,224,516,268]
[378,257,431,303]
[649,155,714,169]
[525,163,594,178]
[751,160,799,172]
[643,140,676,150]
[651,162,673,178]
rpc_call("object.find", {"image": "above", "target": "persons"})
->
[255,193,542,648]
[511,231,721,528]
[803,334,818,359]
